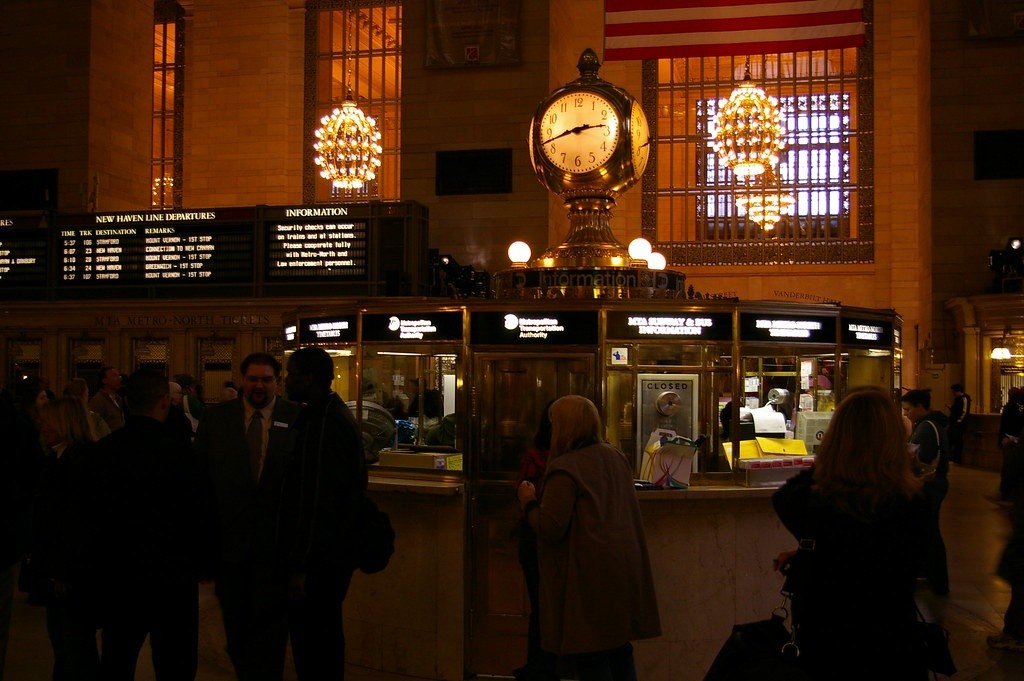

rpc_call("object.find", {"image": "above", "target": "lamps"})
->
[734,164,794,233]
[712,56,785,176]
[990,344,1011,359]
[647,252,666,271]
[313,2,383,189]
[507,241,531,268]
[628,237,653,267]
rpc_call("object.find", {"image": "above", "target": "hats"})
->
[410,377,428,389]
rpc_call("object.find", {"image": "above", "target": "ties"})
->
[245,410,262,489]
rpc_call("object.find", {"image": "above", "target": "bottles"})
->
[1004,433,1021,447]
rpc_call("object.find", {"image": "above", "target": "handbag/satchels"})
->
[334,506,395,573]
[704,607,809,681]
[917,621,957,677]
[904,420,941,482]
[640,428,696,489]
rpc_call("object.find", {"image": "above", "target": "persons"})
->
[771,388,929,681]
[989,389,1024,651]
[0,345,369,681]
[900,390,949,595]
[822,355,848,396]
[391,377,431,419]
[513,395,663,681]
[947,384,971,465]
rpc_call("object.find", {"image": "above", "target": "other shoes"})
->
[987,633,1024,653]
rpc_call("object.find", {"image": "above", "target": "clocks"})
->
[527,47,653,268]
